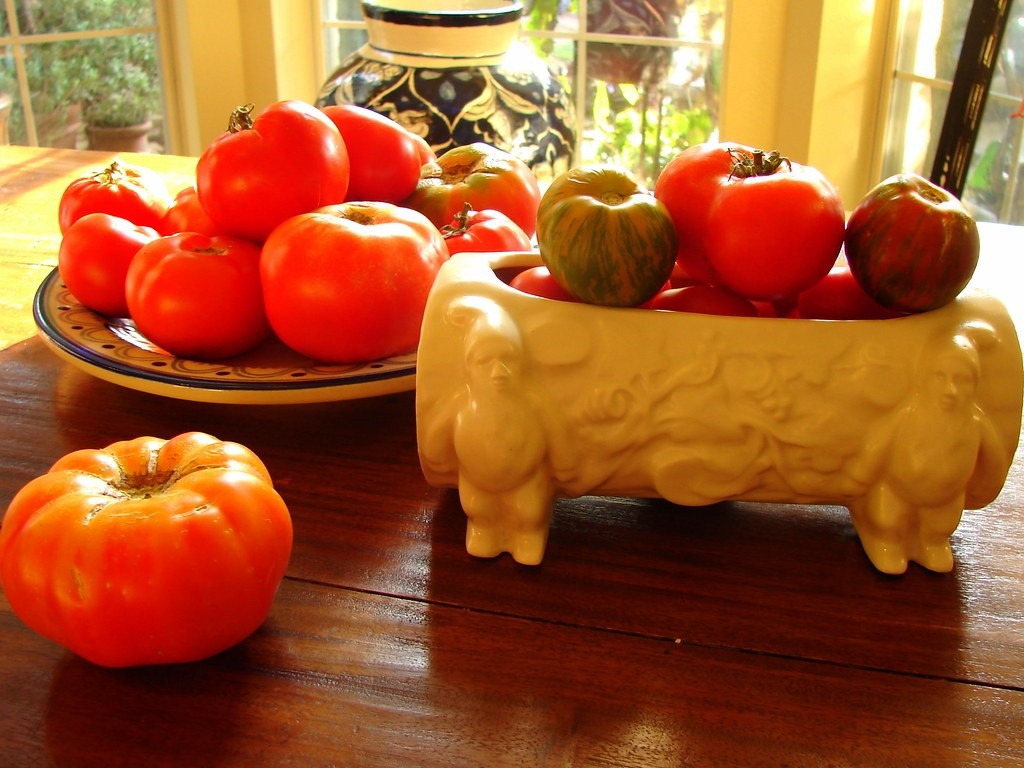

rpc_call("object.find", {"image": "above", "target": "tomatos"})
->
[0,430,293,668]
[511,141,982,320]
[58,101,541,365]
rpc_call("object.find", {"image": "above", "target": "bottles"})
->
[315,0,579,249]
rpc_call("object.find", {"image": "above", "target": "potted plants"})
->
[78,58,152,151]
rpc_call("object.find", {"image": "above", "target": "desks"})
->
[0,140,1024,768]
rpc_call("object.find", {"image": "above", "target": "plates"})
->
[32,265,416,406]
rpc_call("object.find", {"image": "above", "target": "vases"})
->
[321,0,579,192]
